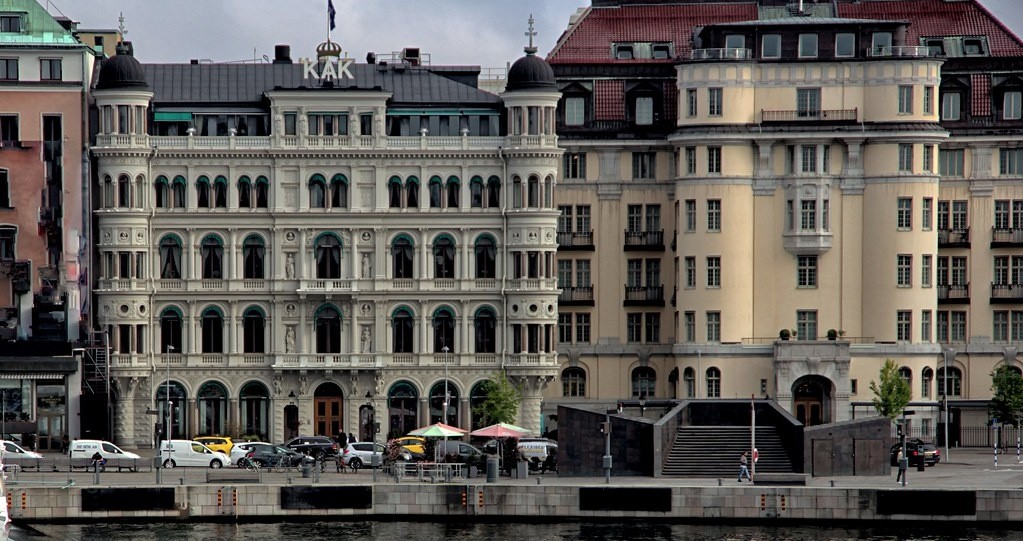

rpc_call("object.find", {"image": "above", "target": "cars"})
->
[338,441,414,469]
[230,442,283,465]
[242,443,316,467]
[397,436,558,472]
[891,441,940,467]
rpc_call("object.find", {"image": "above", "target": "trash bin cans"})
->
[3,463,20,484]
[486,457,500,482]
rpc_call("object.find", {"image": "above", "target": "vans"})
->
[0,440,43,472]
[67,439,131,471]
[192,436,233,456]
[160,439,232,469]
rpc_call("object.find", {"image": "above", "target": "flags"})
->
[328,0,336,31]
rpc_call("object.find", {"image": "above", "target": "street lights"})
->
[166,344,175,440]
[365,390,373,441]
[287,390,296,440]
[442,346,449,464]
[166,400,173,469]
[943,346,954,464]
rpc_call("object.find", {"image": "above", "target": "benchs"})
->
[206,472,262,484]
[754,473,812,487]
[2,457,153,473]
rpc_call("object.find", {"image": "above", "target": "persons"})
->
[896,447,909,485]
[738,452,751,482]
[286,327,298,353]
[244,429,555,477]
[286,254,295,279]
[26,435,104,473]
[362,327,372,353]
[362,254,372,279]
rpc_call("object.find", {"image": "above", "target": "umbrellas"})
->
[407,421,531,476]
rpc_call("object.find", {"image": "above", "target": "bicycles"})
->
[243,450,362,474]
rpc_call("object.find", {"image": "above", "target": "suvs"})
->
[281,435,339,461]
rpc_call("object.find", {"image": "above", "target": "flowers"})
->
[386,438,403,460]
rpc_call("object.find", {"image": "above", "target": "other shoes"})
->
[738,480,742,482]
